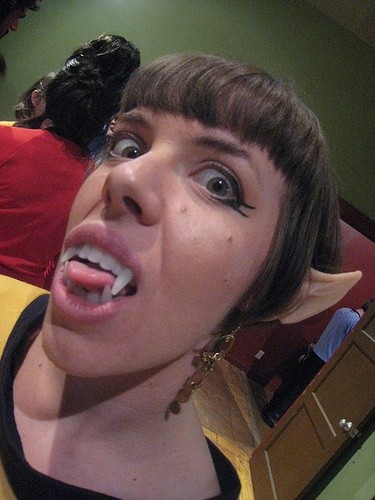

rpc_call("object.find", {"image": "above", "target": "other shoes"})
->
[261,402,281,429]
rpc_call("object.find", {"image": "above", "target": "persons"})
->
[0,52,363,499]
[1,33,143,295]
[0,73,60,131]
[0,0,41,85]
[259,295,375,428]
